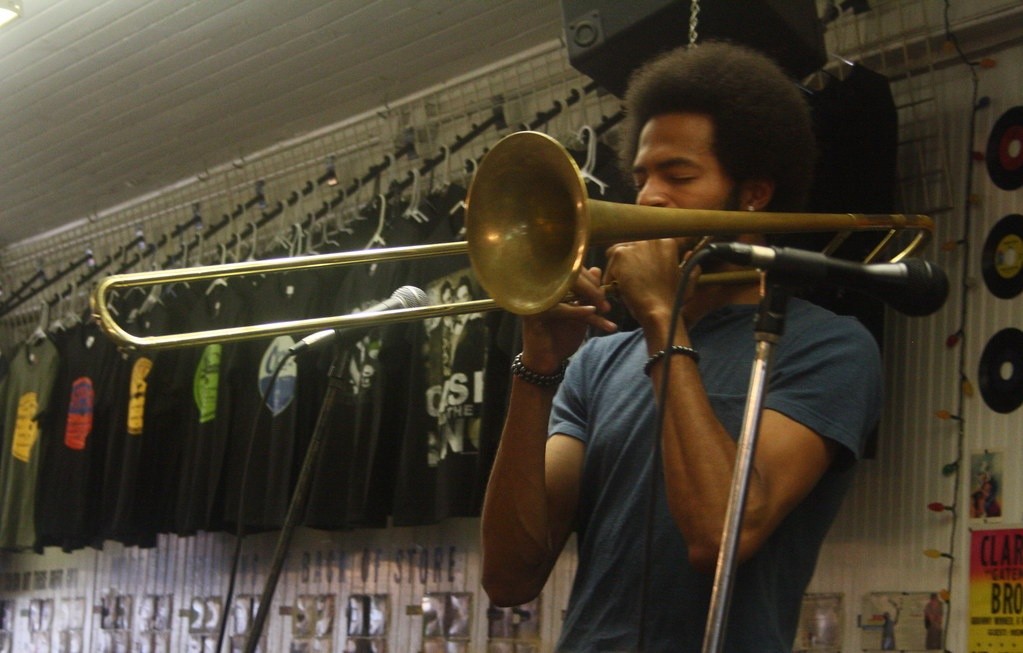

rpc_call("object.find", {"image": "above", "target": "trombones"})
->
[90,130,937,352]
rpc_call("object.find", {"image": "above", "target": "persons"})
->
[427,275,481,459]
[478,41,888,653]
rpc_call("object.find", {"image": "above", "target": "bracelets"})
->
[643,345,700,376]
[511,352,566,386]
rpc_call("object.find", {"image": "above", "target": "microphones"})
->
[289,285,429,355]
[709,243,949,317]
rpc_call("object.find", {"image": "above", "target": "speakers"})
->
[562,0,828,102]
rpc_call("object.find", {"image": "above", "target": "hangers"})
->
[25,85,608,341]
[798,0,854,93]
